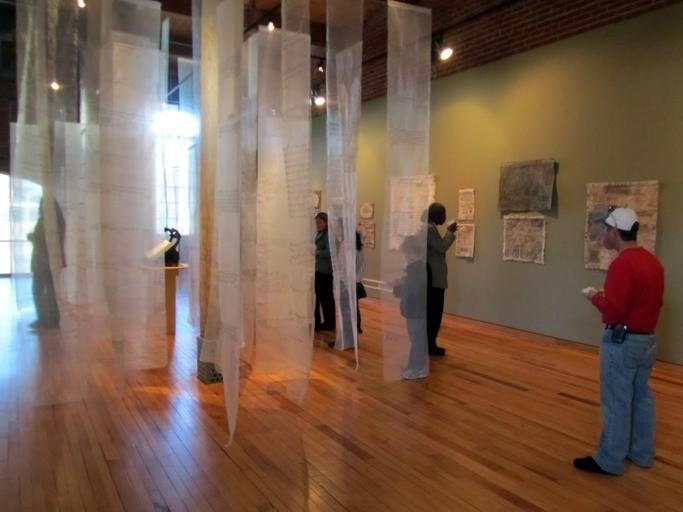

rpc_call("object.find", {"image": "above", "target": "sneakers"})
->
[428,347,445,358]
[575,457,624,475]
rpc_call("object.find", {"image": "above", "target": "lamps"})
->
[306,92,326,107]
[438,43,454,62]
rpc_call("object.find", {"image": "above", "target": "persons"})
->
[26,194,68,329]
[572,204,665,478]
[415,200,459,356]
[308,210,335,332]
[328,215,367,349]
[385,234,433,381]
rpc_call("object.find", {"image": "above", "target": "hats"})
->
[596,208,638,231]
